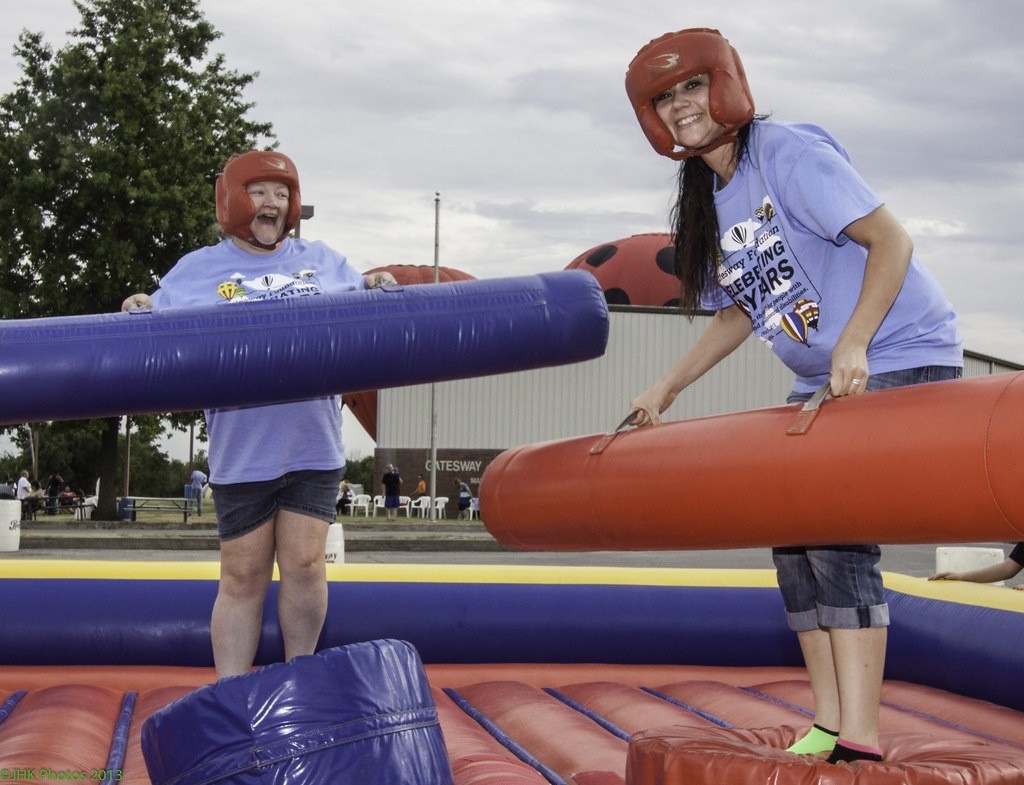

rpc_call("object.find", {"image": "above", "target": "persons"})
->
[928,540,1024,584]
[187,465,208,517]
[57,485,78,513]
[17,470,32,520]
[115,149,399,687]
[454,477,475,522]
[31,481,46,507]
[380,463,404,521]
[44,469,64,507]
[624,26,963,763]
[410,473,427,517]
[336,477,351,516]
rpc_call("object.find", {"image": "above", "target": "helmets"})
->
[625,31,755,160]
[216,148,302,252]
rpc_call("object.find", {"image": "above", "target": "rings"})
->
[852,379,862,385]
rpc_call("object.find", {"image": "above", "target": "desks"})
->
[125,496,191,523]
[25,495,93,522]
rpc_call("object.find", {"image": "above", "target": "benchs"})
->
[29,505,91,511]
[123,507,196,512]
[127,505,195,510]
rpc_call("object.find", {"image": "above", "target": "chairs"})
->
[409,496,431,519]
[458,498,480,521]
[345,490,355,517]
[426,497,449,520]
[372,495,386,517]
[398,496,412,518]
[351,494,371,517]
